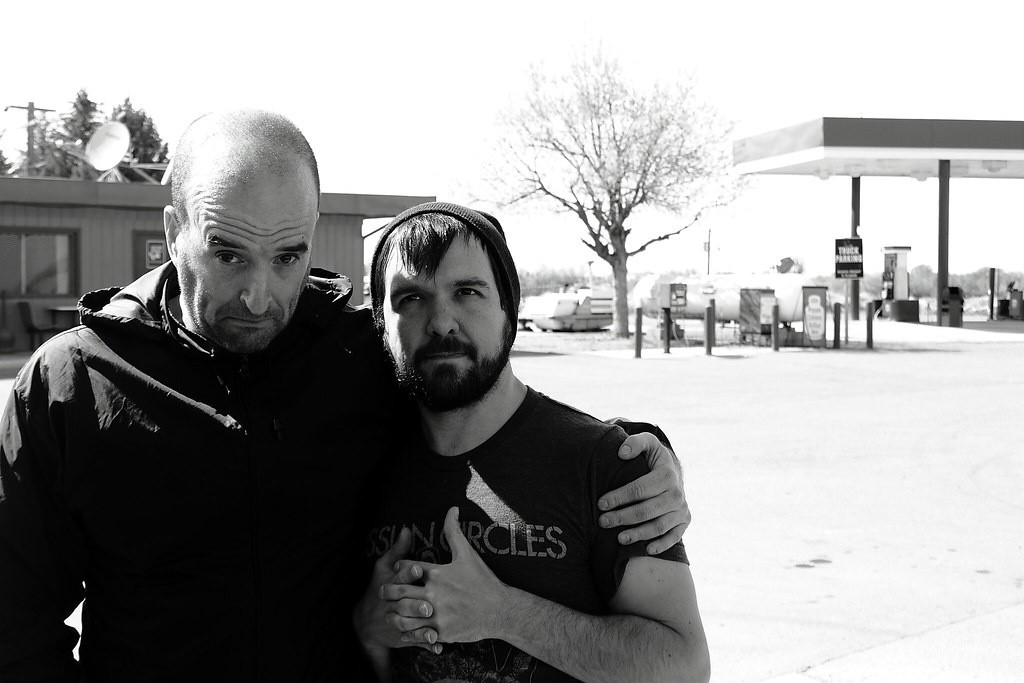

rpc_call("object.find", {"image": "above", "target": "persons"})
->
[0,107,691,683]
[362,202,710,683]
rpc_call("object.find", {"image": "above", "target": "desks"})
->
[45,306,79,328]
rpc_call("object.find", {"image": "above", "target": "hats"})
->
[368,202,521,346]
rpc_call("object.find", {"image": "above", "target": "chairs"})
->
[17,301,68,352]
